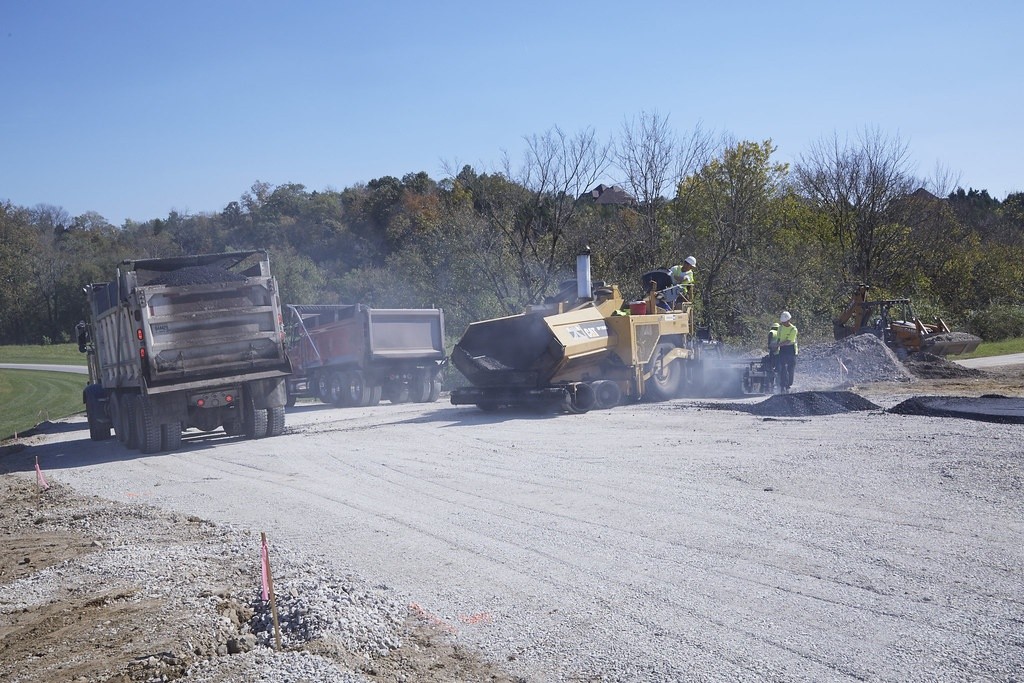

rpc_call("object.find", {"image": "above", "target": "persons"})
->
[657,256,696,311]
[773,311,798,394]
[768,322,781,387]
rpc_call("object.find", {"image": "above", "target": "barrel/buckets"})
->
[630,301,647,314]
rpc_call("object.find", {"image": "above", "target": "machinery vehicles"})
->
[832,283,984,357]
[449,244,778,415]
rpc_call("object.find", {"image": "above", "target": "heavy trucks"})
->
[74,248,286,455]
[284,301,446,406]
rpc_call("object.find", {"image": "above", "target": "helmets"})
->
[772,323,779,327]
[685,256,696,267]
[780,311,791,322]
